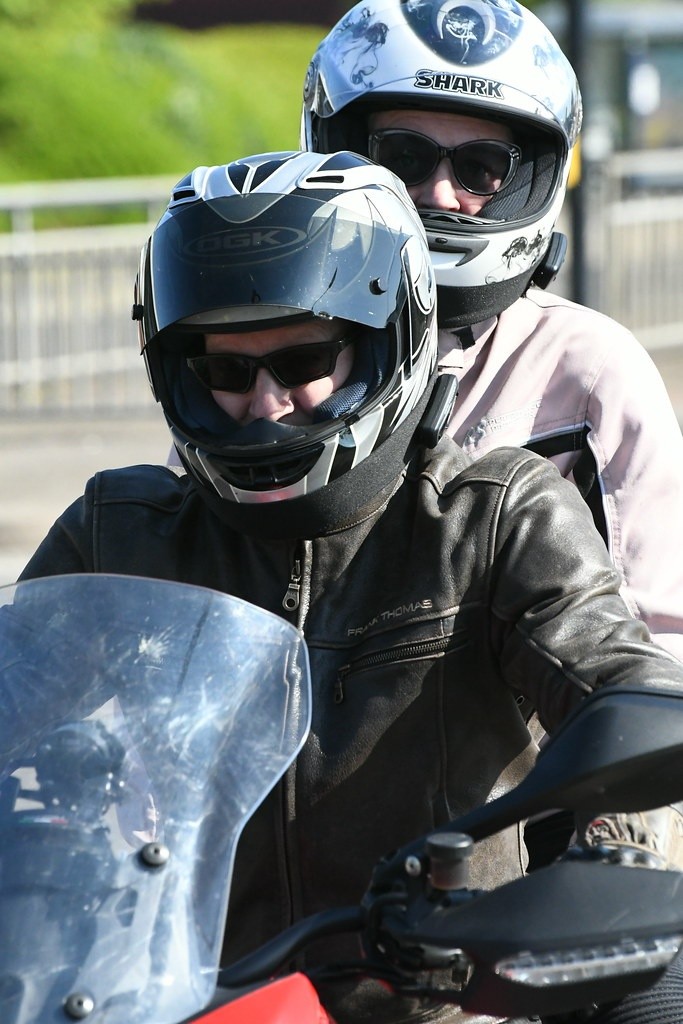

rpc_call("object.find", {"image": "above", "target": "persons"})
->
[7,152,683,1024]
[260,0,683,1024]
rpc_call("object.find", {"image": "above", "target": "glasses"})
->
[186,334,355,393]
[359,128,522,196]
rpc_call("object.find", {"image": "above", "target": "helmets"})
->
[132,149,459,543]
[298,0,585,328]
